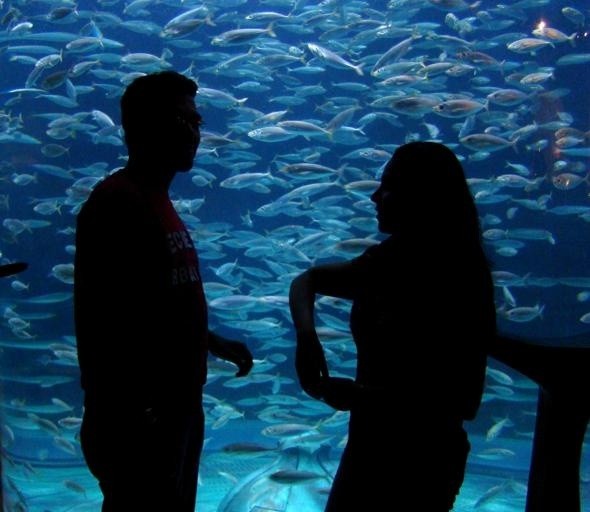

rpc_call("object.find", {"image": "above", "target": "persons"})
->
[288,141,497,512]
[72,70,255,512]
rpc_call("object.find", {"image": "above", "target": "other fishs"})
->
[0,0,590,512]
